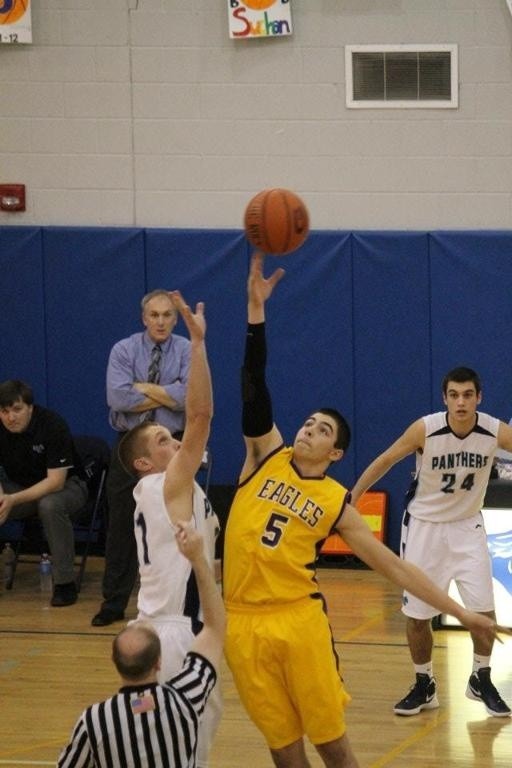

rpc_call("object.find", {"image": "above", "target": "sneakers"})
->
[466,668,511,717]
[394,672,441,716]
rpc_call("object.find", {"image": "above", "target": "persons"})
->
[221,249,512,768]
[54,519,228,768]
[90,290,193,627]
[0,379,91,607]
[116,290,221,768]
[347,365,512,717]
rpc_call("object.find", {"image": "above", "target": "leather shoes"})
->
[51,581,76,606]
[91,608,125,627]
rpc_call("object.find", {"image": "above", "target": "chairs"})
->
[194,446,212,499]
[5,431,111,595]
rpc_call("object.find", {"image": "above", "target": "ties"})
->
[148,346,163,423]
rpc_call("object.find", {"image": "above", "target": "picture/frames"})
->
[0,1,35,46]
[226,0,295,41]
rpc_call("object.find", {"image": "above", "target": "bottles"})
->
[40,553,52,590]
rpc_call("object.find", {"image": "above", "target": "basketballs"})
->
[243,186,310,255]
